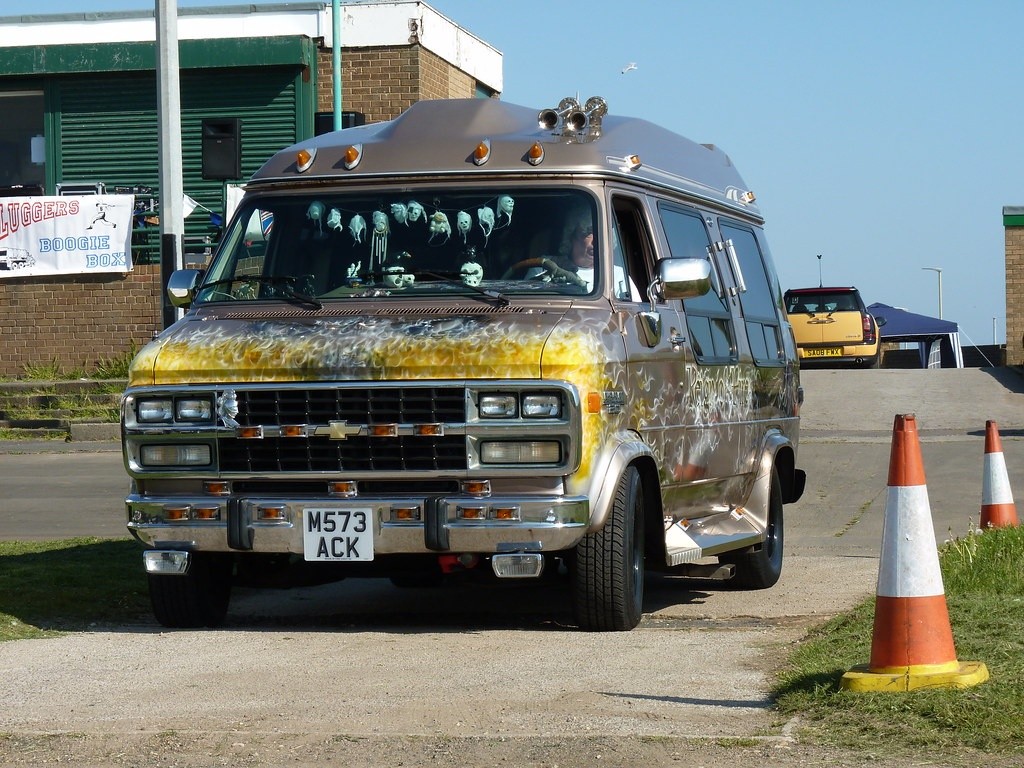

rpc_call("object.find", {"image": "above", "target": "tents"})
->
[866,302,963,369]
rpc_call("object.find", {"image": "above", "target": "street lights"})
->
[921,266,943,320]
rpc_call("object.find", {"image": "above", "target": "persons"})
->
[524,199,642,302]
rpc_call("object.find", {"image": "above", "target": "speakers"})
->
[314,111,365,137]
[202,118,243,180]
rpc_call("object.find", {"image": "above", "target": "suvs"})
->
[784,286,888,369]
[113,91,809,632]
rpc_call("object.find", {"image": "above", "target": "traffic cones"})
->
[981,417,1021,534]
[837,414,992,694]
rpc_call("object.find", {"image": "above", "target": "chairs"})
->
[792,304,807,312]
